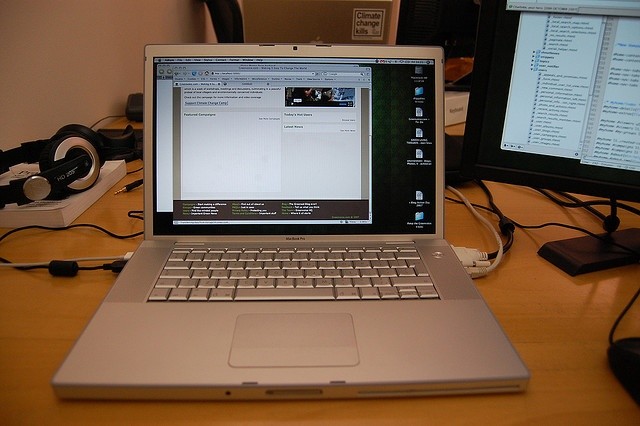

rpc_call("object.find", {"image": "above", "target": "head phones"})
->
[0,123,105,210]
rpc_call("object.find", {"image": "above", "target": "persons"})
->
[292,88,315,101]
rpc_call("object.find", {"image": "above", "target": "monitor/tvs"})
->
[460,1,640,276]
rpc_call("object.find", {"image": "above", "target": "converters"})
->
[125,93,144,121]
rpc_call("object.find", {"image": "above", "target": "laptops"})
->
[50,43,530,400]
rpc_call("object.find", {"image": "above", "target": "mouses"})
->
[606,336,640,402]
